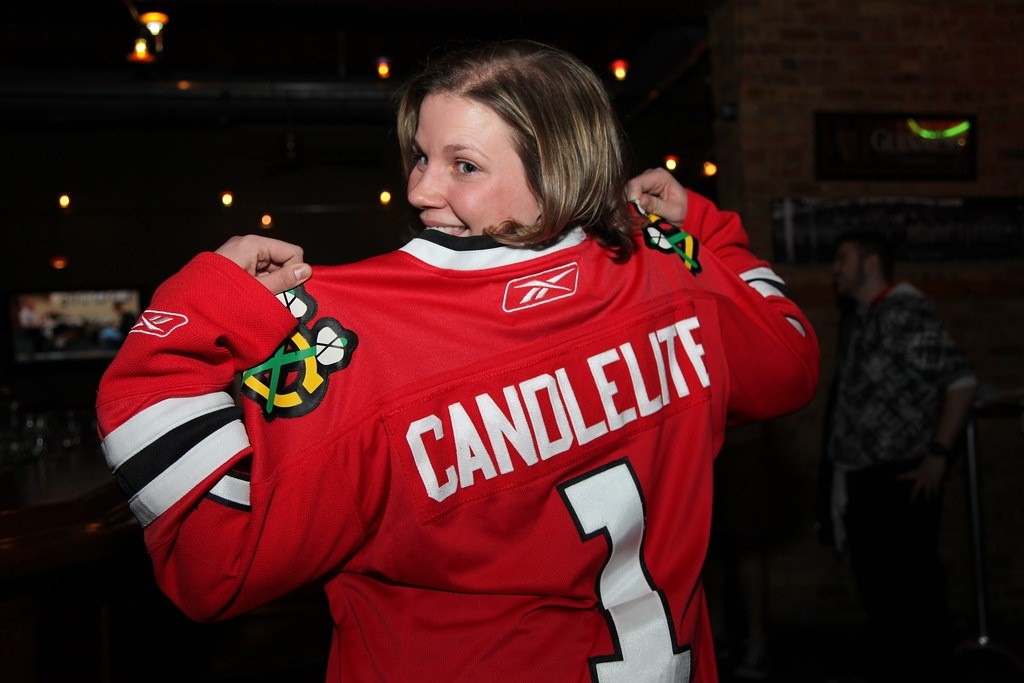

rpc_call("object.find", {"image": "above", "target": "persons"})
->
[105,301,136,348]
[830,227,975,683]
[702,422,813,678]
[94,40,822,683]
[44,313,98,349]
[10,296,54,352]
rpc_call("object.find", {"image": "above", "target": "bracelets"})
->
[927,442,948,456]
